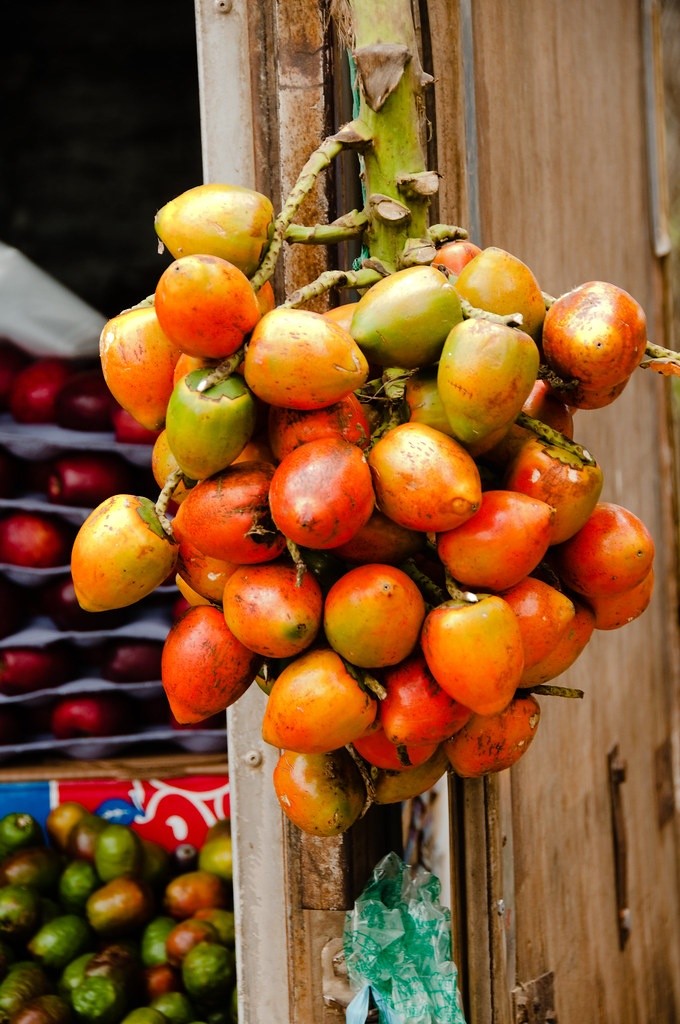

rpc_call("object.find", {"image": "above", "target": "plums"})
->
[1,351,186,744]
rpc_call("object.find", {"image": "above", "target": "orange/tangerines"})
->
[1,802,237,1024]
